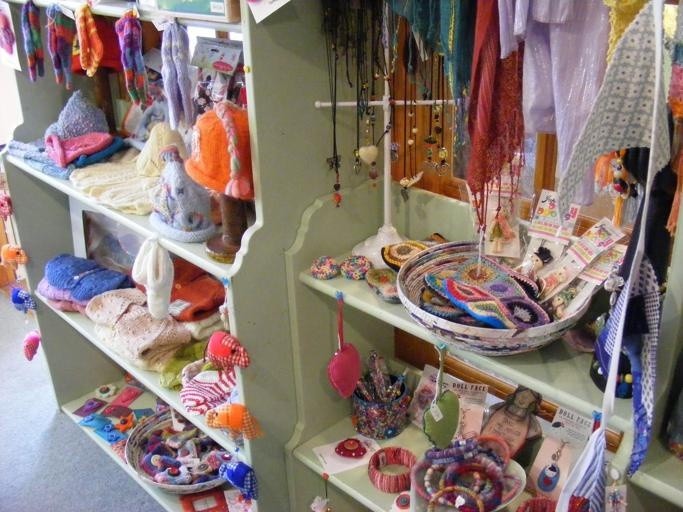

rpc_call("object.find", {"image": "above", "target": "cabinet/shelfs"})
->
[0,1,389,512]
[278,173,682,512]
